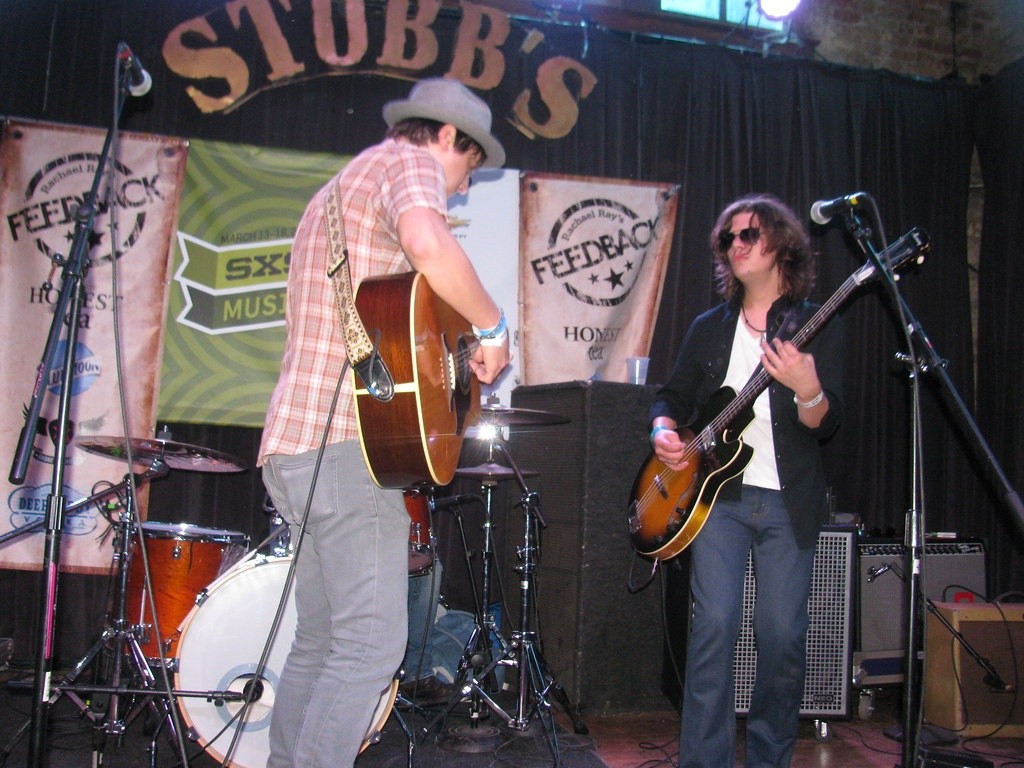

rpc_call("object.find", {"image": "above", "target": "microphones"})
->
[982,675,1014,692]
[810,192,866,225]
[428,494,476,510]
[243,679,264,702]
[118,43,152,96]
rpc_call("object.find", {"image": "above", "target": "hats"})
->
[382,77,507,169]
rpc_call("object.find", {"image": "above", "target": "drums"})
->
[267,501,296,560]
[400,486,437,580]
[102,518,250,670]
[173,552,400,768]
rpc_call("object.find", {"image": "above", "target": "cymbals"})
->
[474,403,572,428]
[455,462,541,481]
[75,435,250,473]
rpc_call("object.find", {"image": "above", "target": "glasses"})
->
[718,228,762,253]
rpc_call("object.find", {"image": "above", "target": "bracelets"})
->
[472,307,507,346]
[651,426,668,447]
[794,390,824,407]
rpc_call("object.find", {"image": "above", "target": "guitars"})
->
[349,269,483,490]
[626,224,932,562]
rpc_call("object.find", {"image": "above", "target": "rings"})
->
[668,459,671,464]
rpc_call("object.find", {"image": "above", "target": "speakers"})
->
[686,522,1024,739]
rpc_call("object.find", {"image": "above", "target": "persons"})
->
[649,194,846,768]
[256,79,512,768]
[406,558,459,700]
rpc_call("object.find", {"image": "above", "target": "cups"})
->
[625,356,650,385]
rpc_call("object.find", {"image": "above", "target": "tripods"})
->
[1,445,183,767]
[416,413,590,768]
